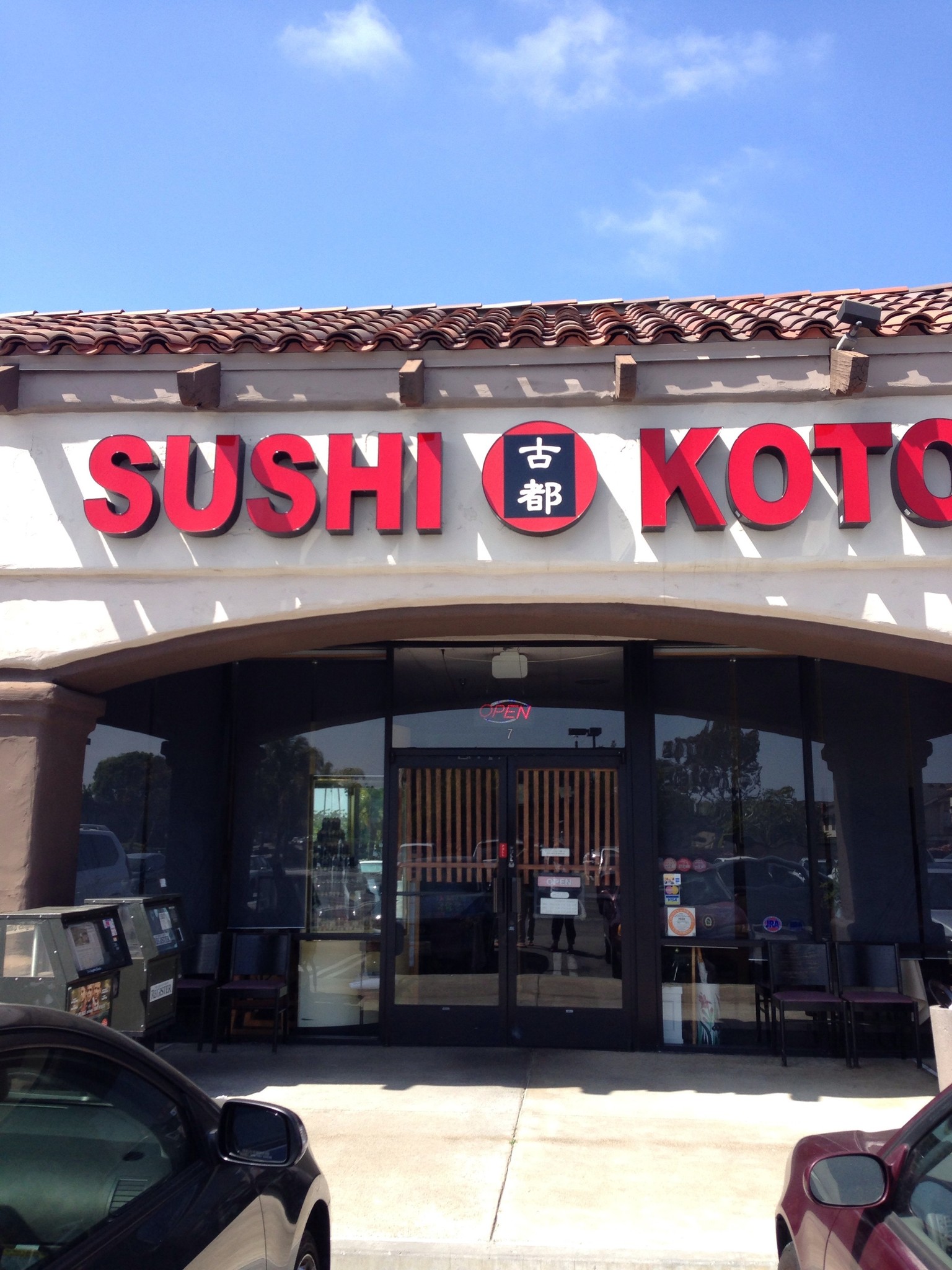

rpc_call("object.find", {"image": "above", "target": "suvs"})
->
[74,824,132,907]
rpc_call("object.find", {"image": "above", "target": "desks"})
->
[665,944,739,1046]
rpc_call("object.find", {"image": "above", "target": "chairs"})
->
[218,925,290,1053]
[754,938,924,1070]
[157,929,221,1052]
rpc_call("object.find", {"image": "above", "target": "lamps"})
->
[568,727,602,748]
[836,299,882,351]
[492,652,528,678]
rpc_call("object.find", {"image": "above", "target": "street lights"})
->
[568,728,587,749]
[587,727,602,748]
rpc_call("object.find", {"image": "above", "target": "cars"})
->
[595,845,952,989]
[127,852,162,895]
[774,1084,952,1270]
[0,1003,334,1270]
[246,838,534,974]
